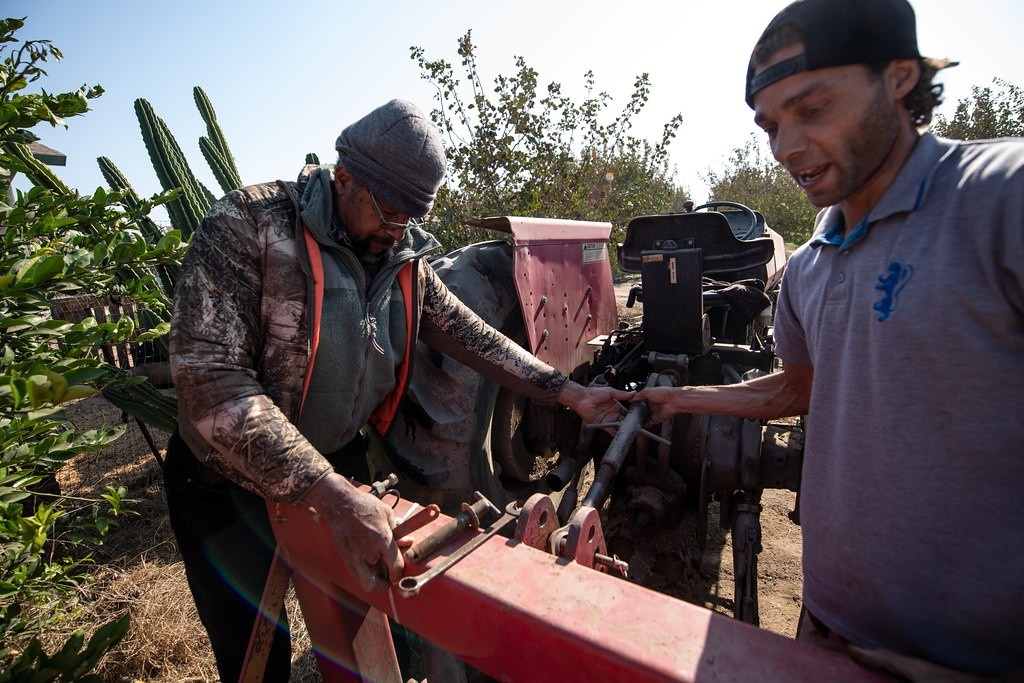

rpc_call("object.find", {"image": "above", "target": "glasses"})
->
[364,188,427,234]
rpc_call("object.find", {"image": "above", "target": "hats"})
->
[333,102,441,219]
[747,0,924,112]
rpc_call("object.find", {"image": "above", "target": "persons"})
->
[625,1,1024,683]
[163,97,637,683]
